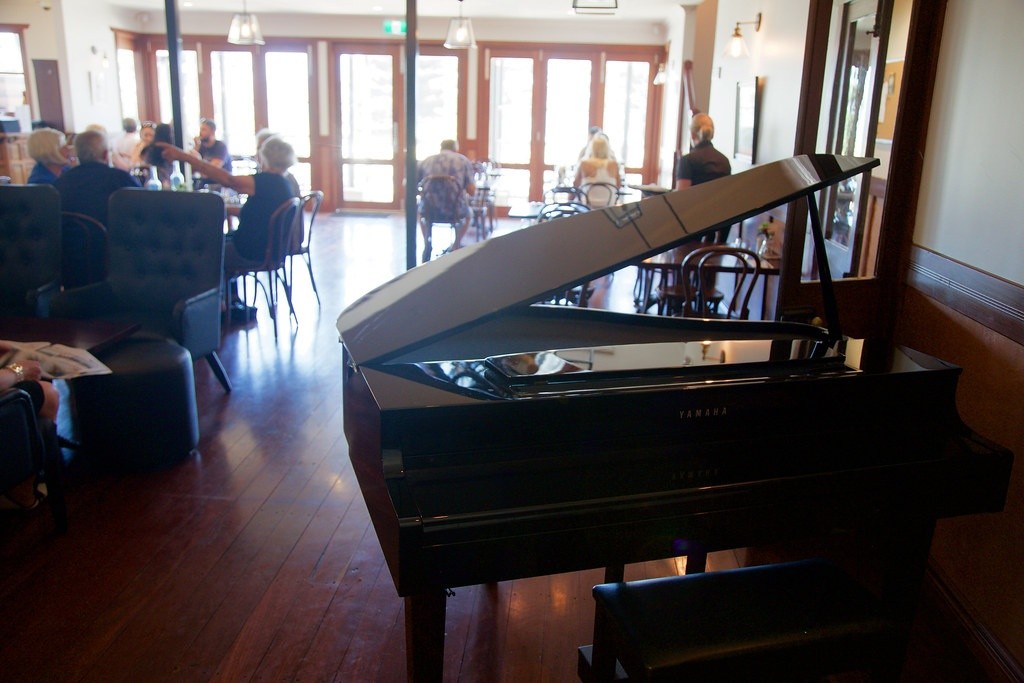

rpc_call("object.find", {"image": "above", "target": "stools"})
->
[86,339,201,470]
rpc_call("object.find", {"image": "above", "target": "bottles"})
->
[144,165,163,191]
[170,160,185,191]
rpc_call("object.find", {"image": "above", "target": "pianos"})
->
[334,151,1016,683]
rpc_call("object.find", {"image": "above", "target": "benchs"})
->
[591,550,916,683]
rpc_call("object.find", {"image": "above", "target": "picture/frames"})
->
[731,74,759,166]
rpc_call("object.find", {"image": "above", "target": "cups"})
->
[0,176,11,184]
[221,186,239,205]
[735,239,750,262]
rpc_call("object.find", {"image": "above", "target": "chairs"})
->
[0,183,323,530]
[418,156,503,256]
[527,181,760,318]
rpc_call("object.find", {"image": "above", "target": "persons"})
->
[155,137,305,310]
[140,123,176,190]
[570,127,623,204]
[668,111,733,192]
[55,132,144,226]
[111,117,144,174]
[418,136,475,261]
[241,128,305,251]
[140,122,157,146]
[25,128,75,185]
[0,357,59,513]
[187,118,234,192]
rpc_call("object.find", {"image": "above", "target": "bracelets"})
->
[8,362,24,382]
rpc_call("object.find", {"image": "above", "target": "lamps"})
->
[653,62,669,85]
[226,0,266,46]
[731,14,761,57]
[443,0,479,49]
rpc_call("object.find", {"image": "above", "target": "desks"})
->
[476,178,490,240]
[0,318,142,456]
[508,202,589,219]
[638,246,774,272]
